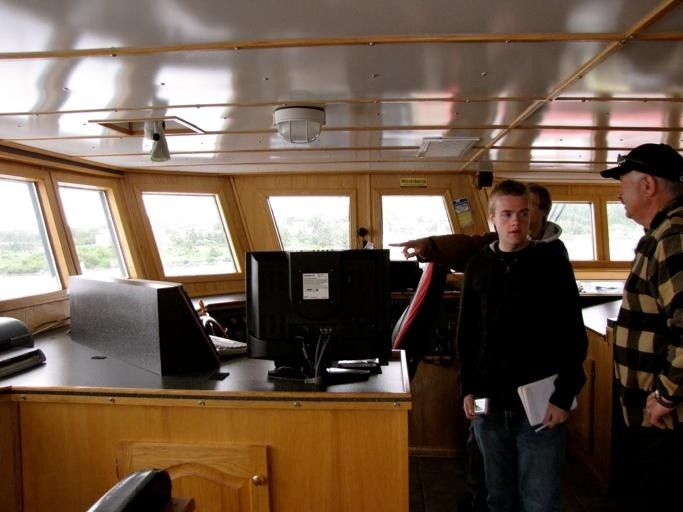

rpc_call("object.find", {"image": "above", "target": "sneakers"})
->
[470,493,485,508]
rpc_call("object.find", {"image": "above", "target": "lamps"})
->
[272,105,325,144]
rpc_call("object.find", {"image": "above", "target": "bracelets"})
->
[653,388,675,409]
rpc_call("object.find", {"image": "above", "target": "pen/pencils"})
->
[596,286,617,289]
[534,421,550,433]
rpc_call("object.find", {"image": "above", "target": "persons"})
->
[598,142,682,511]
[386,182,569,274]
[452,181,585,511]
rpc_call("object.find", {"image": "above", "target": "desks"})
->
[0,292,412,512]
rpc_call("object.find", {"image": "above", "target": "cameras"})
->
[470,398,490,416]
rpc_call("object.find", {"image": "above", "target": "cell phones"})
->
[338,358,379,368]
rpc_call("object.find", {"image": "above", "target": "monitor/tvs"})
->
[245,249,392,384]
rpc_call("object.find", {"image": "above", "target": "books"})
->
[516,374,578,428]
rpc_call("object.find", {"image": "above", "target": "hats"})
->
[602,144,682,183]
